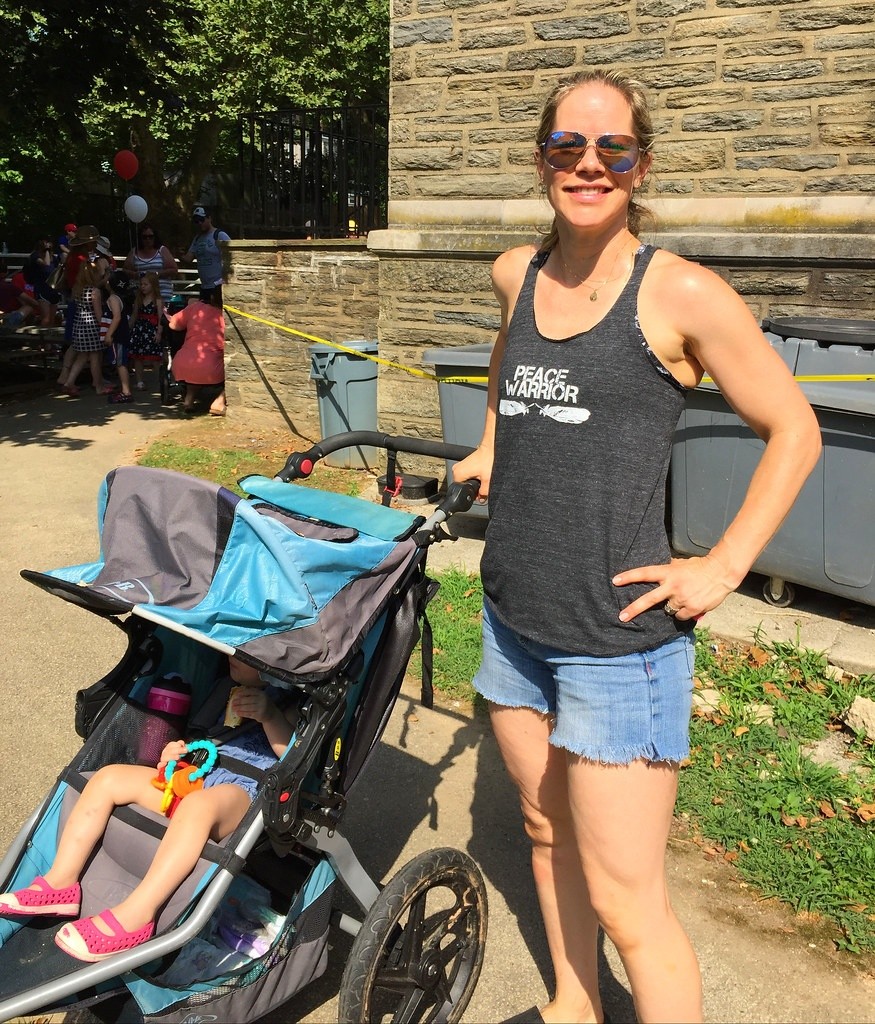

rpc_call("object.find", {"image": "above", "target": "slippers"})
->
[498,1006,612,1024]
[210,405,227,414]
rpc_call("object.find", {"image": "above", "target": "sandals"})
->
[0,874,156,962]
[109,393,134,404]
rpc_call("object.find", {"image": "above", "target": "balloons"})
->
[125,195,147,224]
[115,150,137,180]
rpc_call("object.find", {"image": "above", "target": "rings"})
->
[665,601,679,615]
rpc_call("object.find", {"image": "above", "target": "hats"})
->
[68,225,98,247]
[96,235,112,258]
[192,207,210,217]
[65,223,78,232]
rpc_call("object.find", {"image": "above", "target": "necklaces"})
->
[559,235,637,301]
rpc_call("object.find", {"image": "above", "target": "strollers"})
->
[157,296,189,405]
[1,431,488,1024]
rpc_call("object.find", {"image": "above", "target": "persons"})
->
[62,261,112,396]
[174,206,231,302]
[129,272,164,390]
[448,72,823,1024]
[0,652,292,965]
[8,232,56,328]
[100,271,133,403]
[163,285,224,417]
[96,236,116,285]
[123,228,178,302]
[57,225,116,388]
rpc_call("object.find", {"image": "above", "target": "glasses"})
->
[140,234,155,240]
[540,132,644,174]
[194,216,204,223]
